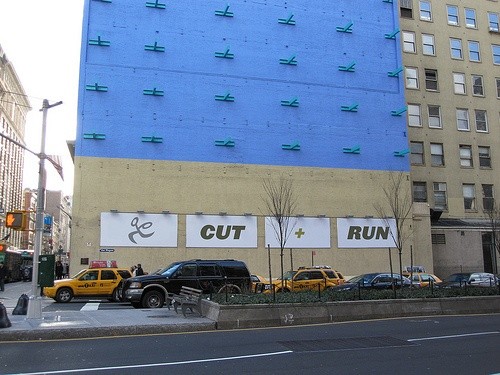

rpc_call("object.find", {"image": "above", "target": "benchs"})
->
[167,286,203,319]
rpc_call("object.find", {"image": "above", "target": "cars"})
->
[431,273,499,291]
[328,273,419,295]
[402,273,443,288]
[251,274,267,293]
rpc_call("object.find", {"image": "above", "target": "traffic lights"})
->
[6,212,23,228]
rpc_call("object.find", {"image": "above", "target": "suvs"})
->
[255,266,345,294]
[116,260,252,308]
[41,268,133,303]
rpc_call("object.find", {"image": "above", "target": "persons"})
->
[129,263,144,277]
[0,258,69,292]
